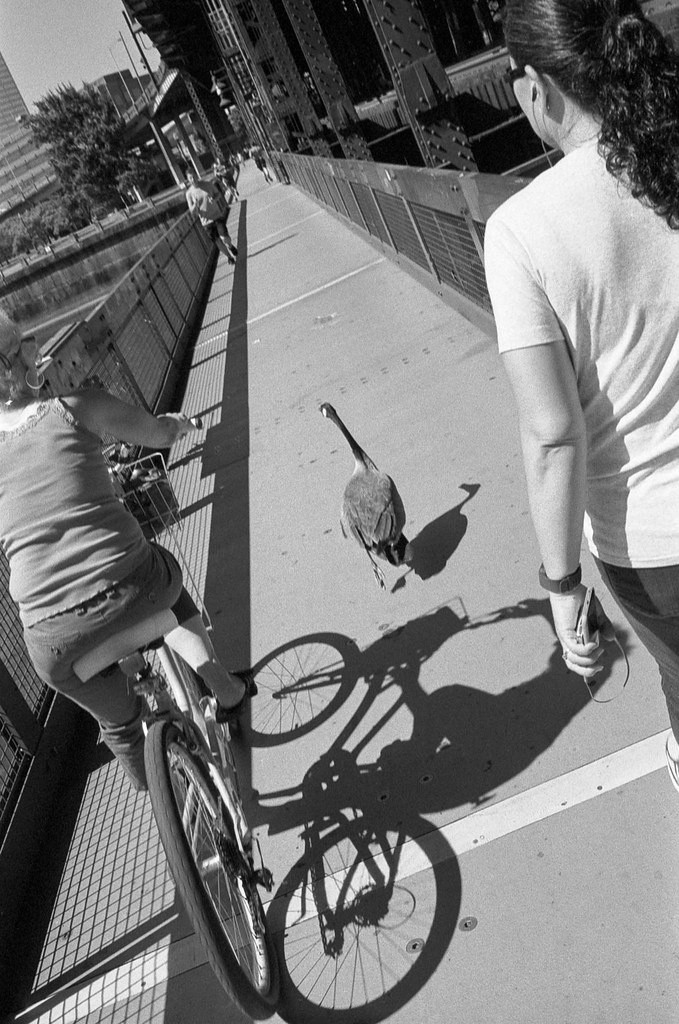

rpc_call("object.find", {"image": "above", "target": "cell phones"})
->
[581,586,600,645]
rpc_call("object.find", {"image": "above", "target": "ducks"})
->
[316,400,404,590]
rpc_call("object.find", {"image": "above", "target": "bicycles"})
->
[84,419,286,1021]
[225,592,563,1023]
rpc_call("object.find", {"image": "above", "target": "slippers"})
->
[215,669,253,723]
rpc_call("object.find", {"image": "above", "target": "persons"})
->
[248,141,273,182]
[229,144,250,168]
[212,157,239,201]
[0,306,257,824]
[483,1,679,791]
[185,168,238,264]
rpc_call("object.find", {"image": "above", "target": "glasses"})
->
[16,336,36,355]
[504,65,544,83]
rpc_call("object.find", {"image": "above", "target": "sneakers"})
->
[665,731,679,791]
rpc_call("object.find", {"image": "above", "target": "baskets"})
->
[101,441,186,542]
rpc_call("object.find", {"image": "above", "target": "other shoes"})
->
[230,245,238,255]
[227,256,234,265]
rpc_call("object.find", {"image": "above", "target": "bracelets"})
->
[539,563,582,593]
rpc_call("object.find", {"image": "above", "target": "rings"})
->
[562,650,568,660]
